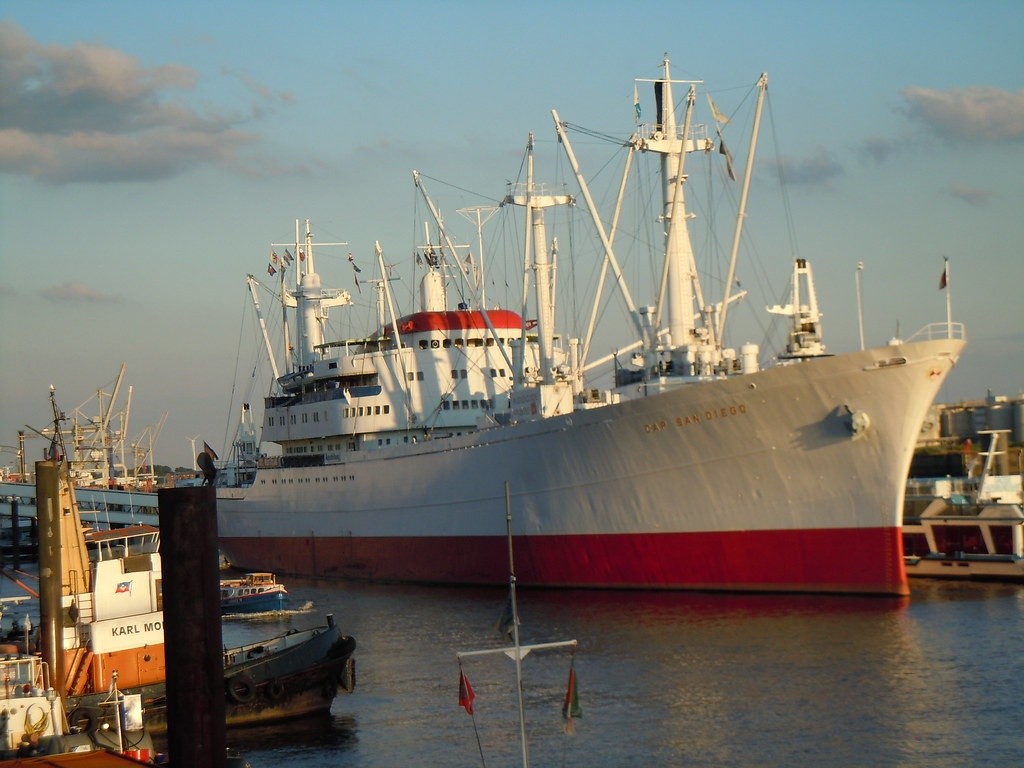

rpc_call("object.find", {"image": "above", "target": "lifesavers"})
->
[267,678,285,699]
[431,340,439,348]
[229,673,255,704]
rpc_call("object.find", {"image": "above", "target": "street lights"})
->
[856,261,865,349]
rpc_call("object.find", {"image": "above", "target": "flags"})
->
[458,670,475,716]
[633,86,641,118]
[268,263,277,277]
[707,96,731,125]
[938,268,946,290]
[271,250,279,265]
[299,248,305,261]
[562,656,582,718]
[281,249,294,279]
[719,141,738,182]
[348,252,362,294]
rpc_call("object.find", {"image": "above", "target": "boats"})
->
[219,573,290,615]
[215,58,966,598]
[64,526,357,736]
[0,596,164,768]
